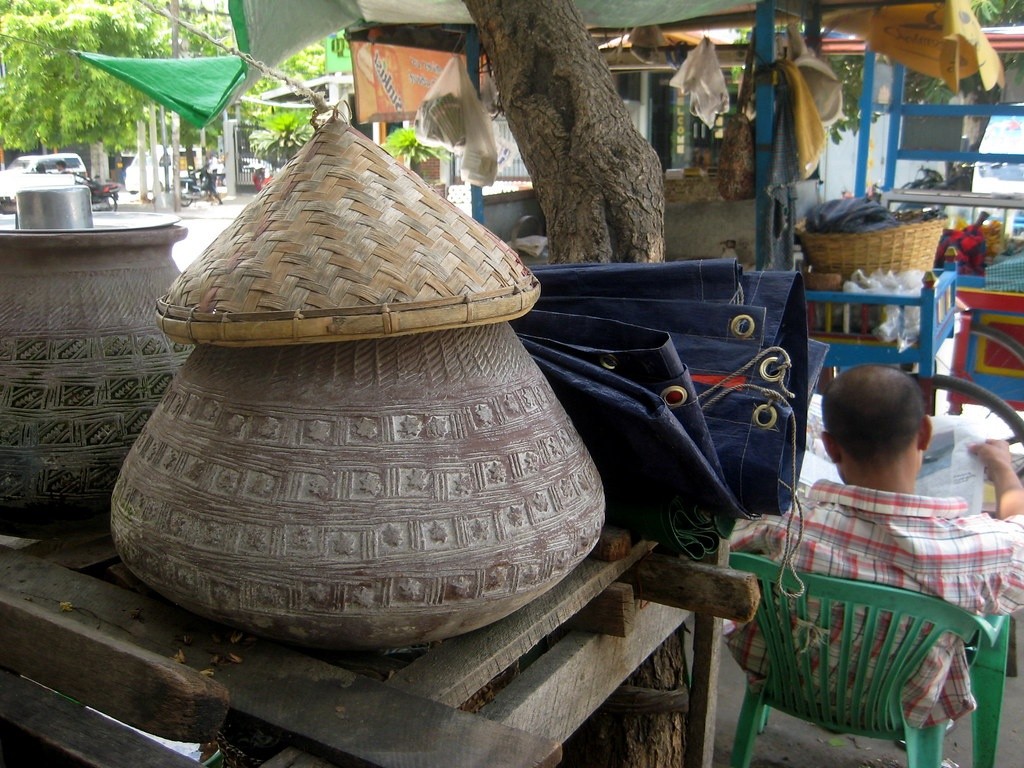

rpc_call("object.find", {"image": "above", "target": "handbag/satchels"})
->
[414,57,466,156]
[458,57,499,187]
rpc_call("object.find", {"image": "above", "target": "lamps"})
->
[626,23,666,64]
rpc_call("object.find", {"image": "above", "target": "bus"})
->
[179,149,201,178]
[109,155,135,179]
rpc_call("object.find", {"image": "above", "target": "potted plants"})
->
[380,126,452,202]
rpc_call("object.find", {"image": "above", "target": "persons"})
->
[36,164,52,174]
[723,364,1024,751]
[207,155,223,205]
[56,160,70,174]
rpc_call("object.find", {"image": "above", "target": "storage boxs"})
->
[0,226,196,522]
[107,122,606,651]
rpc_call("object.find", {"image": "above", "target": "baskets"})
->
[794,210,948,274]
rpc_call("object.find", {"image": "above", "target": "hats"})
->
[153,100,542,347]
[210,150,216,155]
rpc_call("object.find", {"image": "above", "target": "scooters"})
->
[244,164,272,191]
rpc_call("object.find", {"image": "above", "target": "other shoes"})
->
[218,201,223,204]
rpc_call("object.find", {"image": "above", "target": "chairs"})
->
[728,552,1010,768]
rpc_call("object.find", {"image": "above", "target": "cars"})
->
[125,148,173,195]
[0,153,87,214]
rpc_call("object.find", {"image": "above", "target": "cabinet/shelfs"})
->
[854,39,1024,416]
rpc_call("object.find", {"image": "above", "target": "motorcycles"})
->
[76,174,121,212]
[179,159,223,207]
